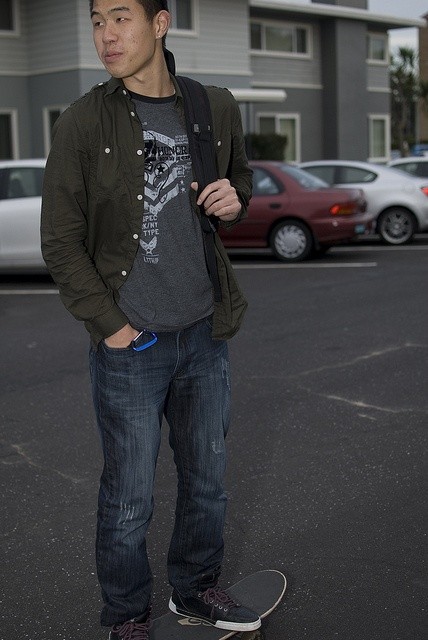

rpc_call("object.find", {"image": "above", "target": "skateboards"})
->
[109,569,287,640]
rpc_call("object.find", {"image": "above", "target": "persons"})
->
[40,0,261,640]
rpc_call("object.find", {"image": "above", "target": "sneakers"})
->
[109,611,151,639]
[168,588,261,631]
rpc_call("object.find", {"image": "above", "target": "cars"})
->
[258,160,428,246]
[0,158,54,282]
[355,157,428,233]
[218,160,366,262]
